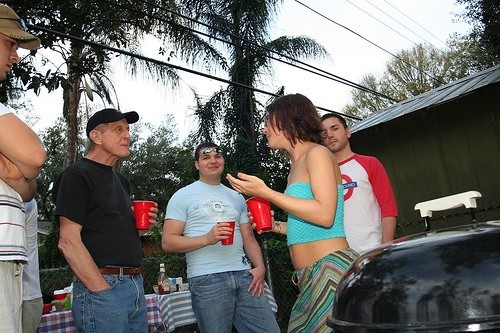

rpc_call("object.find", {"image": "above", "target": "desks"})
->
[34,296,167,333]
[145,279,278,333]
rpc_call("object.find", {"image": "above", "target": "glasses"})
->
[0,18,28,32]
[199,147,223,155]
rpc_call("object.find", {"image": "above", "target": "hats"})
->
[86,108,139,138]
[0,3,41,50]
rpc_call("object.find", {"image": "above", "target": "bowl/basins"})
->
[42,303,53,315]
[179,283,189,291]
[152,285,158,294]
[54,293,71,300]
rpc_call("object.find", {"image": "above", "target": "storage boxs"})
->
[169,277,183,285]
[179,283,189,292]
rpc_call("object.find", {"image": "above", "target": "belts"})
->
[98,266,140,275]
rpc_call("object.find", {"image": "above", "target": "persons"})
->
[318,113,398,256]
[56,108,149,333]
[162,142,282,333]
[226,93,360,333]
[0,2,47,333]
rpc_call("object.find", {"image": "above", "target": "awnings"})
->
[346,64,500,135]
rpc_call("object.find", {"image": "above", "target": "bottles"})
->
[158,263,170,295]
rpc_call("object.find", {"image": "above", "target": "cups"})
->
[245,195,273,232]
[217,220,235,245]
[133,200,156,230]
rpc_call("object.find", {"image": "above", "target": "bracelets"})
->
[274,221,281,234]
